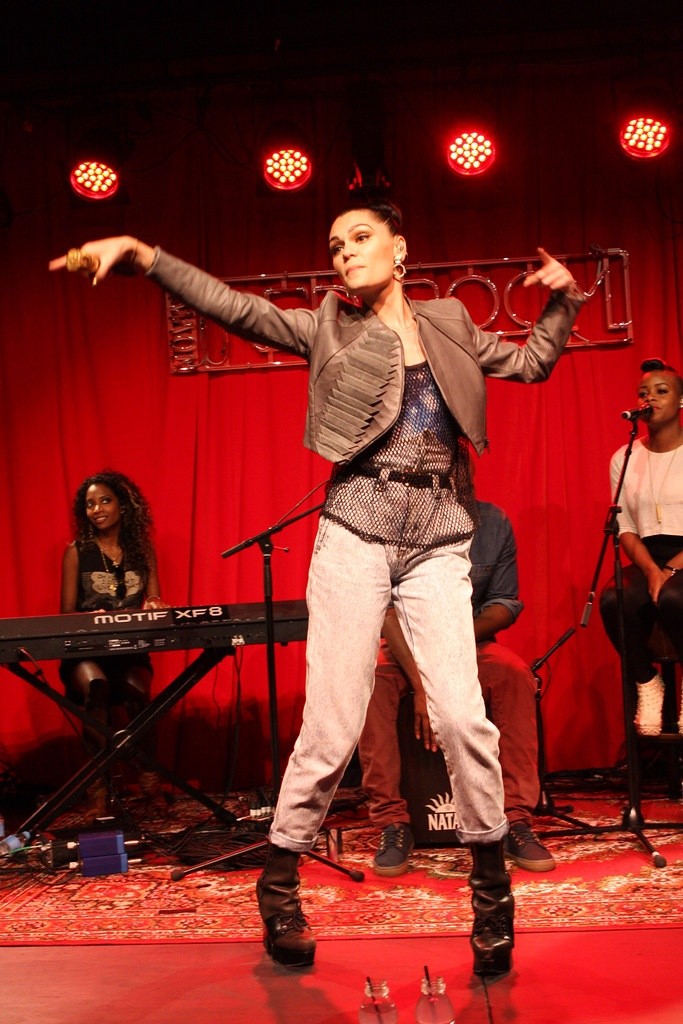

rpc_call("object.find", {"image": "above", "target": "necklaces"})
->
[383,319,415,333]
[97,539,123,572]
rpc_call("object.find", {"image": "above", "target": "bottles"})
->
[415,975,455,1024]
[358,977,398,1024]
[0,831,30,857]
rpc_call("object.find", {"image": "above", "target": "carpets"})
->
[0,782,683,949]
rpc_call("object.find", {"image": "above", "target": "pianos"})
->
[0,597,309,847]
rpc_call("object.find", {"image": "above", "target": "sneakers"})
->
[373,826,414,877]
[504,823,555,871]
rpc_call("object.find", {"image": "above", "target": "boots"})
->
[139,772,168,819]
[255,839,316,967]
[468,840,514,974]
[85,778,110,826]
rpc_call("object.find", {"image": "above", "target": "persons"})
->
[59,472,164,821]
[600,360,683,736]
[48,198,584,975]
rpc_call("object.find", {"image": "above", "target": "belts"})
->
[352,464,451,489]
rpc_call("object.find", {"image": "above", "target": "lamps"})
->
[258,118,318,196]
[438,106,500,180]
[615,84,677,162]
[68,129,123,205]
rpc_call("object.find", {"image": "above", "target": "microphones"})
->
[621,403,653,419]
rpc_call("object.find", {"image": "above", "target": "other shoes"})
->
[677,679,683,735]
[633,675,665,736]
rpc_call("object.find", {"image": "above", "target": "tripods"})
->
[171,504,366,886]
[526,418,683,869]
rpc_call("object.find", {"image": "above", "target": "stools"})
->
[629,615,683,800]
[398,693,495,848]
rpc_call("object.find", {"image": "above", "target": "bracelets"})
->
[147,595,160,600]
[664,565,681,577]
[131,238,139,263]
